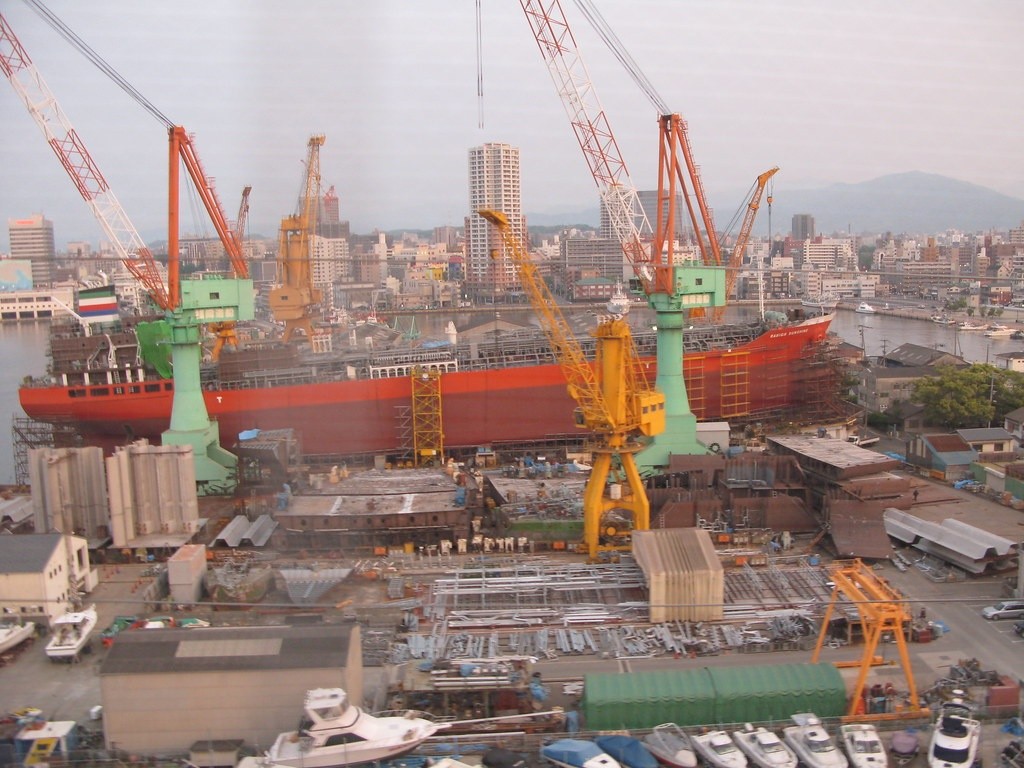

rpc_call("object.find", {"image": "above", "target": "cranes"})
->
[298,157,335,211]
[0,0,253,364]
[268,132,326,348]
[474,0,781,328]
[478,208,666,562]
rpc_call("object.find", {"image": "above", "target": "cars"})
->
[981,601,1024,621]
[1013,621,1023,638]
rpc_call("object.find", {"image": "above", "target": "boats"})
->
[927,712,982,768]
[855,301,877,315]
[595,734,659,768]
[689,727,748,768]
[45,602,100,660]
[984,324,1019,336]
[958,321,990,330]
[930,314,956,325]
[783,717,849,768]
[267,687,453,767]
[841,724,887,768]
[539,737,621,768]
[643,722,698,768]
[733,723,798,768]
[18,308,837,456]
[0,621,36,653]
[606,275,630,315]
[426,758,480,768]
